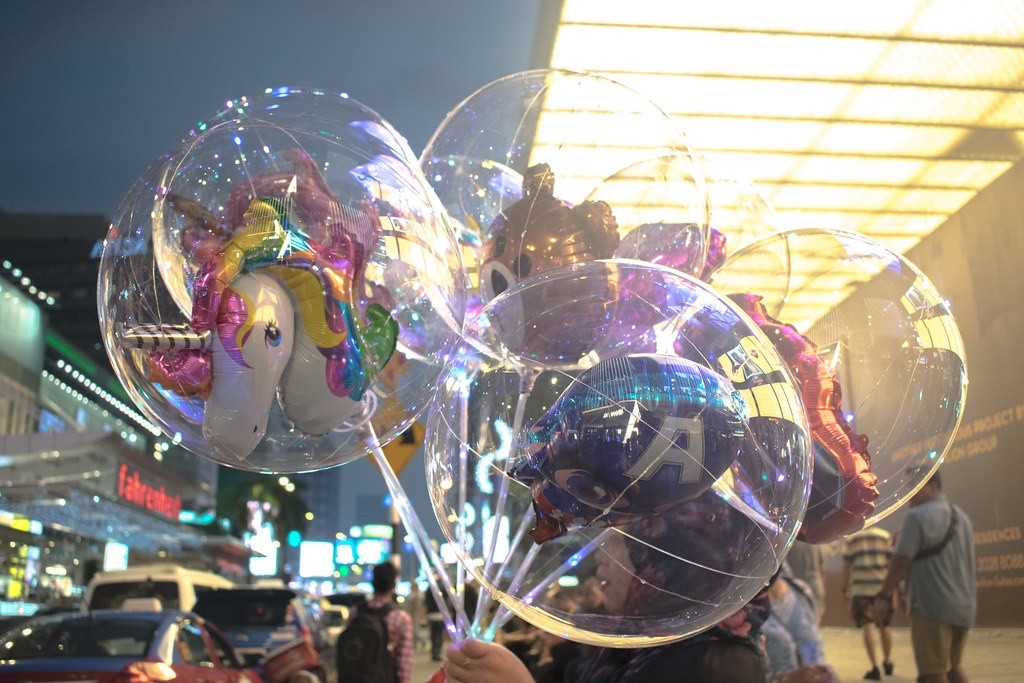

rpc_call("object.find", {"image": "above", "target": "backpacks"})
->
[336,601,400,683]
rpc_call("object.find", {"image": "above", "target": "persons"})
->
[875,465,977,683]
[442,353,769,683]
[281,604,297,626]
[841,508,905,680]
[405,571,604,665]
[349,562,414,683]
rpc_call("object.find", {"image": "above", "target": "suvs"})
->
[182,586,338,683]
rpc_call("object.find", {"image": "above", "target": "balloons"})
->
[97,68,968,650]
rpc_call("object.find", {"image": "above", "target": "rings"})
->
[462,656,471,668]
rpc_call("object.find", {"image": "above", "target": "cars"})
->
[0,606,267,683]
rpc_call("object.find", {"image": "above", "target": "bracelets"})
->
[842,587,849,592]
[876,591,894,602]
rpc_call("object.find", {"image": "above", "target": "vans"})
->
[77,563,239,663]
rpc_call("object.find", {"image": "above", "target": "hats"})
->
[373,561,399,579]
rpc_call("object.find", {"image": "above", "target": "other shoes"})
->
[431,655,443,661]
[883,663,893,676]
[864,666,880,680]
